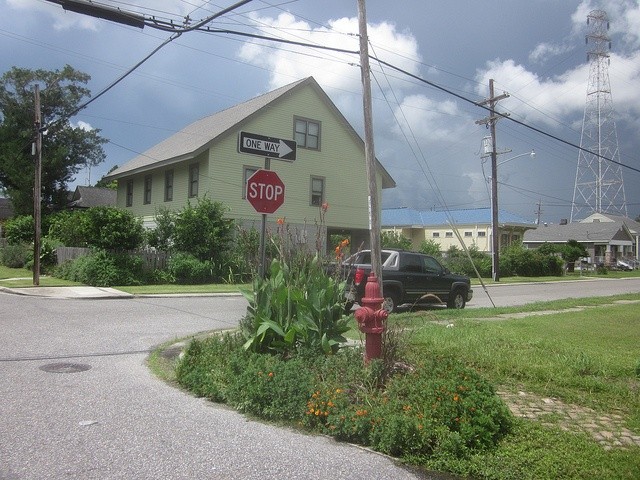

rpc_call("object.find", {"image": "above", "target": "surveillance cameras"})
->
[530,150,537,160]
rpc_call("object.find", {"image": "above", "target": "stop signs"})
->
[245,168,286,215]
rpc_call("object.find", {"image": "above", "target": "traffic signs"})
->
[237,130,297,164]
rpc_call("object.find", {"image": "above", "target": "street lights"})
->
[491,149,536,280]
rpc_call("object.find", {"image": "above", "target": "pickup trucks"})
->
[321,248,474,314]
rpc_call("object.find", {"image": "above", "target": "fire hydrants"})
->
[354,272,389,366]
